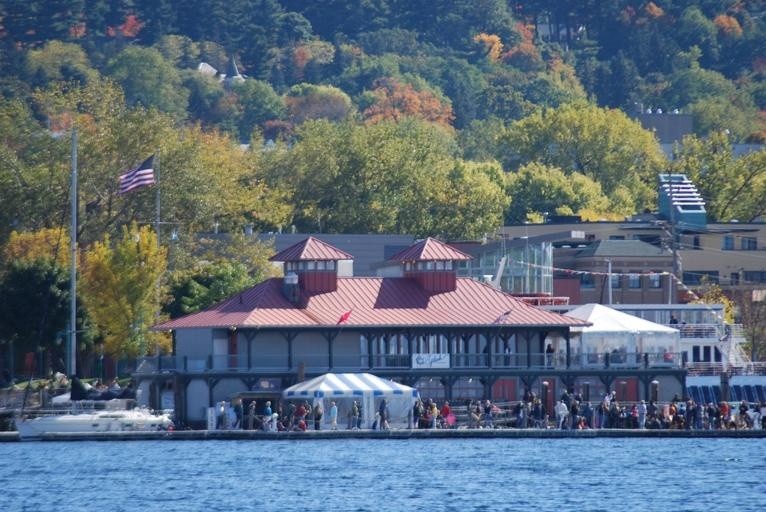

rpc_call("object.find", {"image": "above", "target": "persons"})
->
[467,398,493,428]
[414,398,457,428]
[664,349,674,363]
[515,390,765,430]
[545,342,555,367]
[214,398,392,430]
[669,313,721,336]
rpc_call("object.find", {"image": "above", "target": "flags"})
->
[337,310,352,327]
[115,154,157,195]
[493,310,510,324]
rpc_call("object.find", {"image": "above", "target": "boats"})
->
[16,399,172,439]
